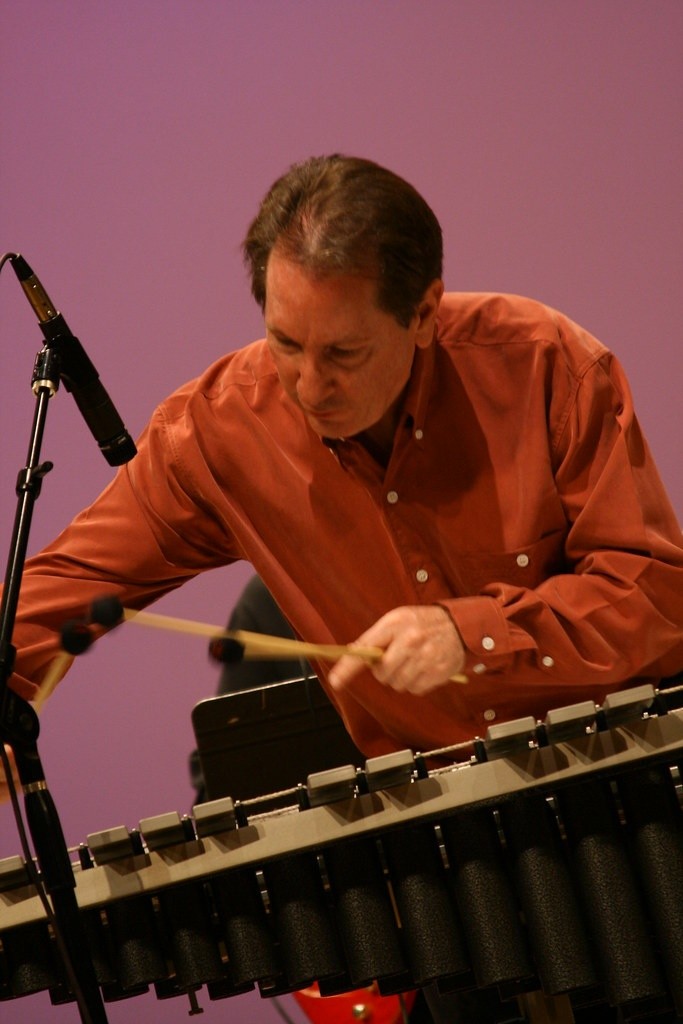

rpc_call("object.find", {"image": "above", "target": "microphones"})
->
[9,252,138,467]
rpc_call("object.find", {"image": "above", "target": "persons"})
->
[0,151,683,1024]
